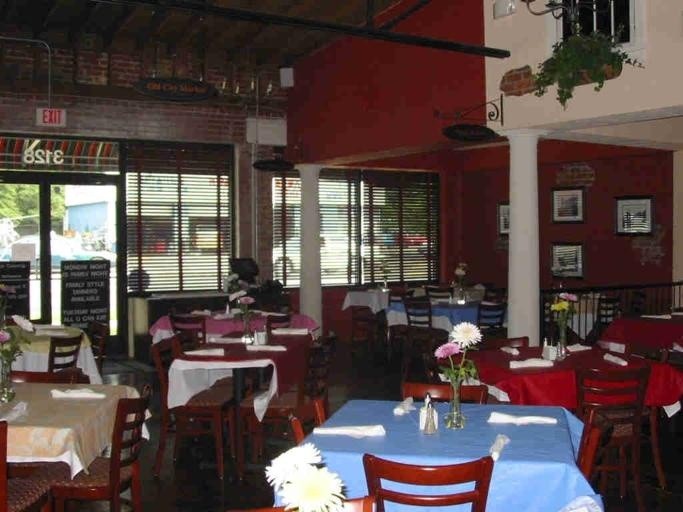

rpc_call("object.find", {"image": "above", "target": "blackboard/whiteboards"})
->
[0,261,30,320]
[61,260,111,334]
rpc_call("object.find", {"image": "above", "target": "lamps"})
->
[252,145,293,173]
[433,92,504,142]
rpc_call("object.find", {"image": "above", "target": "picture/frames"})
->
[551,186,587,226]
[549,240,586,280]
[611,194,656,237]
[496,202,509,237]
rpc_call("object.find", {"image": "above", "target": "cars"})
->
[1,233,119,272]
[380,226,438,261]
[192,224,224,251]
[274,232,379,278]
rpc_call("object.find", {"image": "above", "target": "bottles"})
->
[253,328,259,345]
[225,303,229,315]
[541,337,548,356]
[425,402,436,433]
[557,342,562,359]
[424,394,432,408]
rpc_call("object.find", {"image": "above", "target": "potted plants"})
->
[530,25,643,108]
